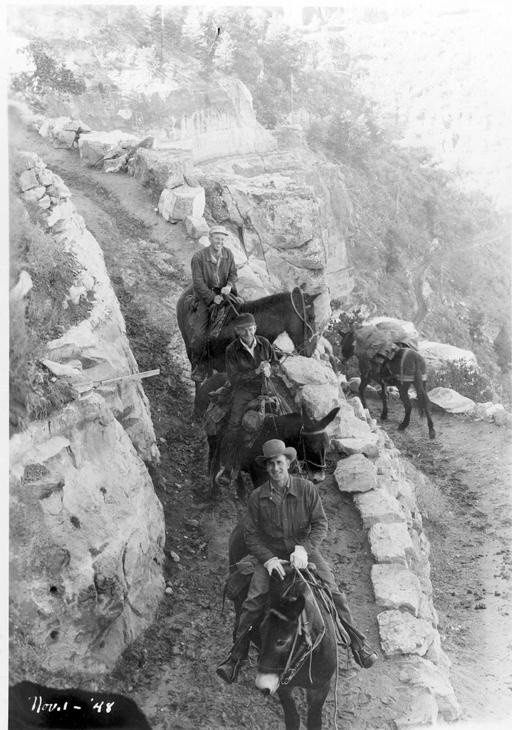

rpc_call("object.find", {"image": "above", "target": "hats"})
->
[208,225,229,236]
[233,313,256,329]
[255,439,298,468]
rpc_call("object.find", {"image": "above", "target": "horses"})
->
[337,328,436,440]
[176,281,322,399]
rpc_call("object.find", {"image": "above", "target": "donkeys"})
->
[228,519,338,730]
[193,372,341,508]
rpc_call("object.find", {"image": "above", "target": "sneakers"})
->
[352,649,378,668]
[216,467,231,485]
[216,662,239,685]
[192,365,206,383]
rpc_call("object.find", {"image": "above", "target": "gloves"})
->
[289,545,308,569]
[263,557,288,581]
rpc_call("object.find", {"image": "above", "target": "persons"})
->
[215,439,378,683]
[218,314,317,485]
[190,225,239,383]
